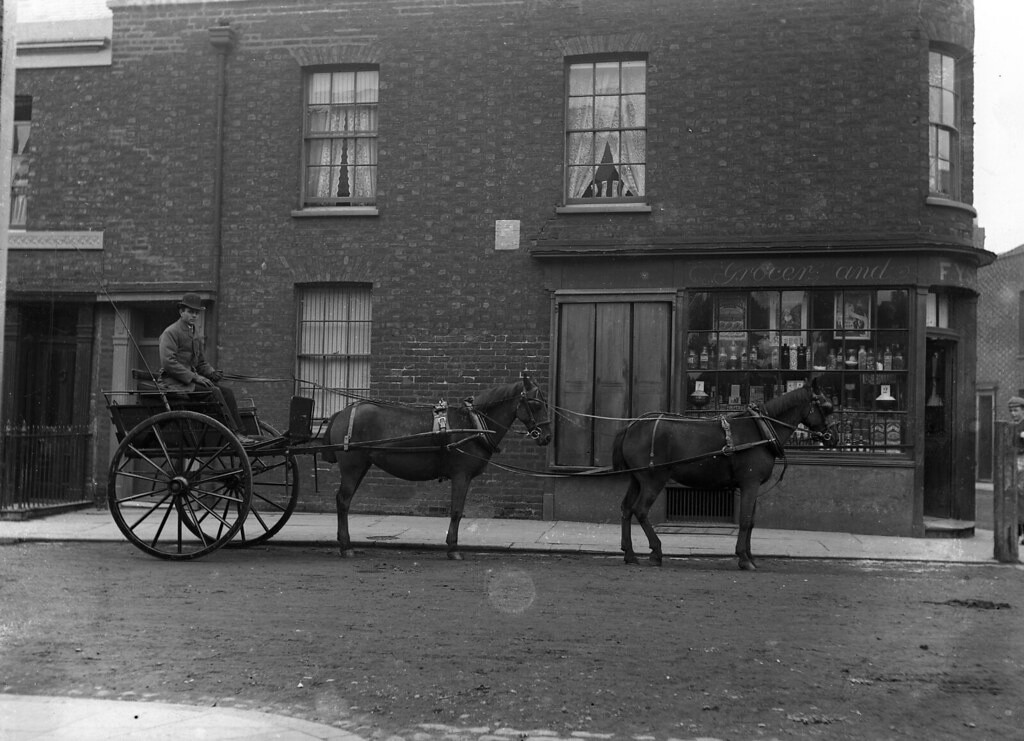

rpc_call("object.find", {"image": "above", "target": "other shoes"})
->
[233,432,255,445]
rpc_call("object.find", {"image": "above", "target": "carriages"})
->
[100,370,835,572]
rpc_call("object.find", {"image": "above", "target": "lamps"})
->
[689,380,708,403]
[876,385,895,403]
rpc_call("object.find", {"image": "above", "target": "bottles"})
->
[821,386,857,409]
[686,341,906,370]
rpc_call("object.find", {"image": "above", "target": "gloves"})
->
[192,374,214,388]
[208,370,224,382]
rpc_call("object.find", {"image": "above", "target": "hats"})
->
[176,293,206,311]
[1008,397,1024,407]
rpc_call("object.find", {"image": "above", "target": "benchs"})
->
[132,368,169,391]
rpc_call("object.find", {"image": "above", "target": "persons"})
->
[1008,397,1024,545]
[158,293,260,446]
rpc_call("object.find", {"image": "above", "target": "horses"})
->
[320,373,553,561]
[611,378,839,571]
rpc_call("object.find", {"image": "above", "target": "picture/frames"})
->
[831,289,877,345]
[709,289,748,345]
